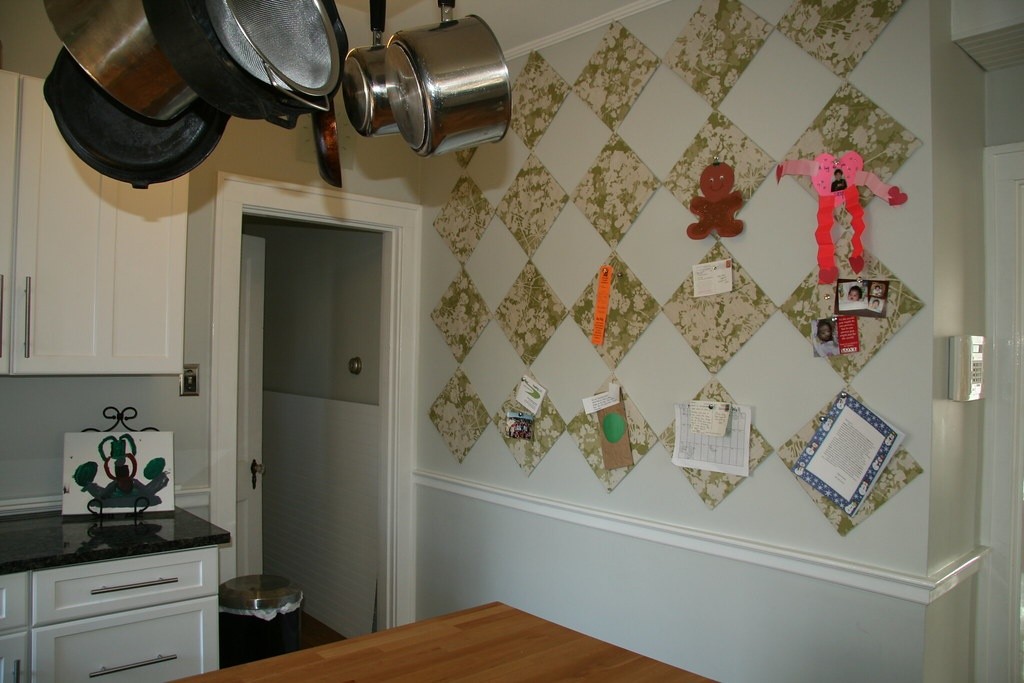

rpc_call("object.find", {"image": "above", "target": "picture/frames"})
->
[179,364,199,396]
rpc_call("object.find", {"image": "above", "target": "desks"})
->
[162,600,721,683]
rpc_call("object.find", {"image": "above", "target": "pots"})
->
[44,0,512,190]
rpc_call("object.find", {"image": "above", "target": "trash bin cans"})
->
[219,574,303,671]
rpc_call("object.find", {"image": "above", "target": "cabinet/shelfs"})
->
[0,69,189,376]
[1,544,220,683]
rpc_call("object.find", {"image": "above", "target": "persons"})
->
[847,285,883,312]
[831,168,847,191]
[506,417,533,440]
[816,321,833,343]
